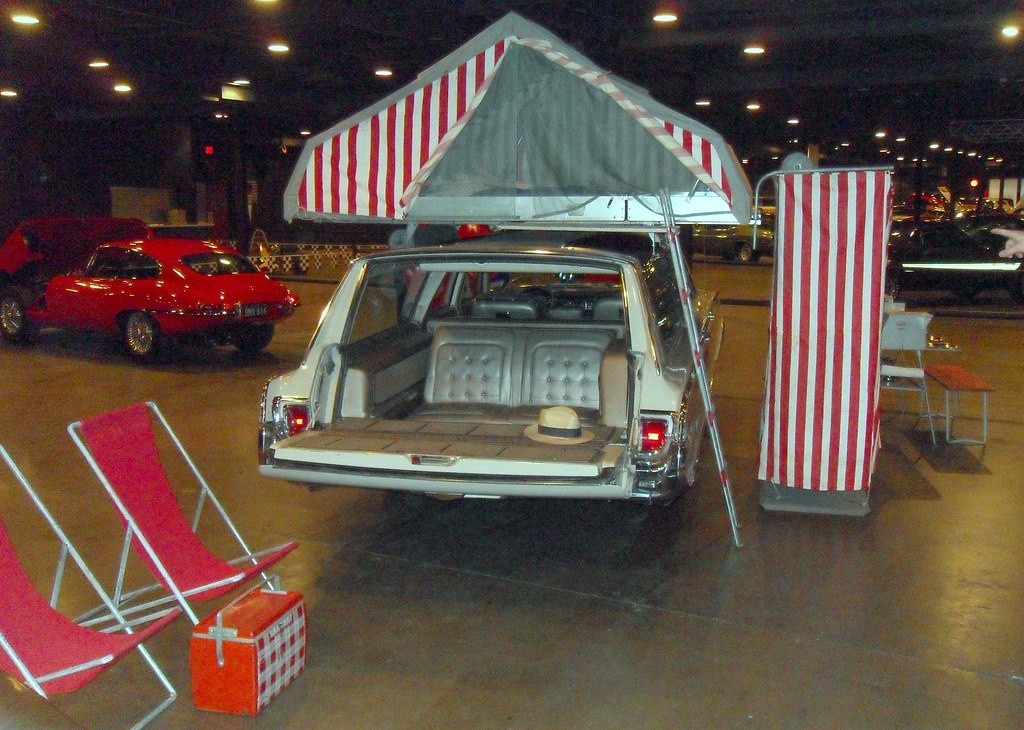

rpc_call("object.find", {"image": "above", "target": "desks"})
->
[879,313,937,445]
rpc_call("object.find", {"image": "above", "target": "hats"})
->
[524,406,595,445]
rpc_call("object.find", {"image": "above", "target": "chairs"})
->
[0,442,178,730]
[71,401,299,627]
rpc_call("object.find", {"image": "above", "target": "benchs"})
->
[918,362,995,445]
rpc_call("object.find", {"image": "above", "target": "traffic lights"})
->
[198,134,224,184]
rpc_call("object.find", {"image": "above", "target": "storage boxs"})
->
[190,574,309,716]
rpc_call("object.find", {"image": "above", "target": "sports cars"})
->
[0,215,300,362]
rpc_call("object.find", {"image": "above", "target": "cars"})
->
[885,216,1023,306]
[691,205,775,265]
[253,225,724,511]
